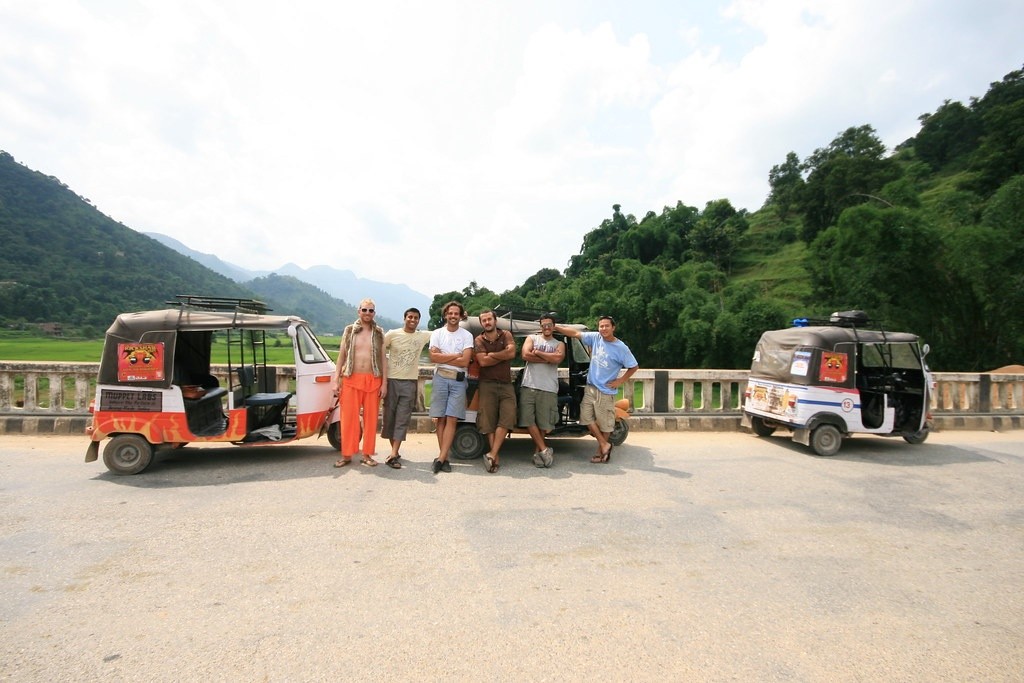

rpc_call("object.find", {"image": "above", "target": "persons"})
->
[519,314,565,468]
[380,308,468,469]
[334,300,388,467]
[536,315,639,464]
[429,301,473,474]
[475,310,517,473]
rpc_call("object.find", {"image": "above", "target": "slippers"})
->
[590,455,601,463]
[385,458,401,469]
[490,462,499,472]
[386,454,401,460]
[483,454,493,471]
[601,442,613,463]
[360,458,377,467]
[334,459,351,467]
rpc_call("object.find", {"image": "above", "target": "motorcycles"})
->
[743,318,938,456]
[442,305,629,460]
[84,294,363,475]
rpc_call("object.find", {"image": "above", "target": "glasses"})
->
[361,309,375,313]
[541,323,554,328]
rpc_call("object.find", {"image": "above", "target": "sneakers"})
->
[540,447,553,466]
[532,453,544,467]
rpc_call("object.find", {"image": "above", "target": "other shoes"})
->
[432,458,443,473]
[441,460,451,472]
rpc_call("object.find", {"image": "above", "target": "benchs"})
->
[247,393,292,405]
[558,395,573,405]
[177,384,229,408]
